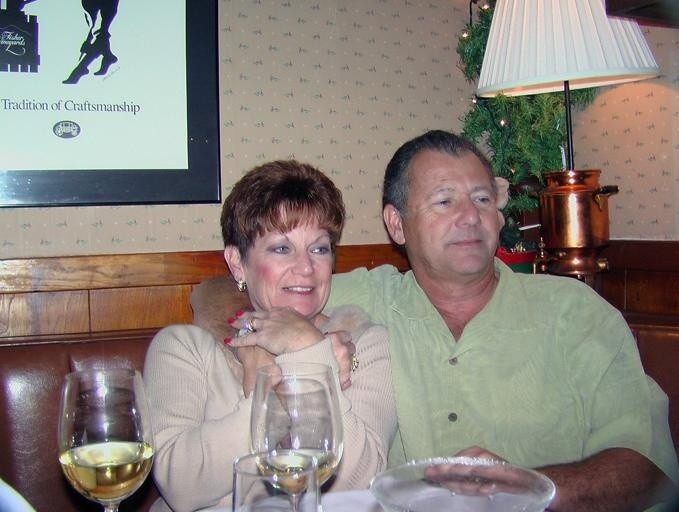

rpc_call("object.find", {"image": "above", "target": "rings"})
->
[244,316,260,334]
[353,355,360,375]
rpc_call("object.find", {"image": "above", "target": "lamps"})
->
[474,0,661,277]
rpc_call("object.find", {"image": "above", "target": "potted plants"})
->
[455,0,598,245]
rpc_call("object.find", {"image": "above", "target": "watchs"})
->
[231,330,255,364]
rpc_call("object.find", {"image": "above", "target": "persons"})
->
[141,161,398,512]
[203,131,679,510]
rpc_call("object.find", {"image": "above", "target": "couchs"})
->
[0,313,675,510]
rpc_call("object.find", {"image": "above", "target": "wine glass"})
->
[250,361,345,512]
[229,454,321,506]
[56,367,156,512]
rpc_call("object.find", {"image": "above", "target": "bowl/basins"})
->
[368,456,557,512]
